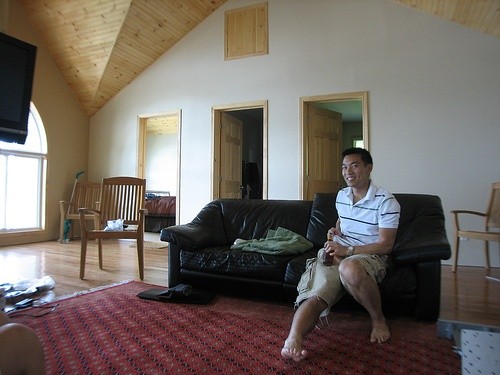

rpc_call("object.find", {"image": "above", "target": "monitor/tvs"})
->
[0,32,37,145]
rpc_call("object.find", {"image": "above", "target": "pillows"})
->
[306,192,339,246]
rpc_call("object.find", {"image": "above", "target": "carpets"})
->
[4,278,462,375]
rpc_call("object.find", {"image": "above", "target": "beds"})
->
[146,190,176,233]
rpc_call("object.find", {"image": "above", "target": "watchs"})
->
[348,246,354,256]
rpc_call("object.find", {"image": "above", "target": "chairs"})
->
[78,177,146,280]
[450,181,500,271]
[59,181,105,244]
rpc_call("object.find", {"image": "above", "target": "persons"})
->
[281,147,401,361]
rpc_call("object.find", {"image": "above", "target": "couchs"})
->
[160,193,452,324]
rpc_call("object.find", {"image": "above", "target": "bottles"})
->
[323,233,333,266]
